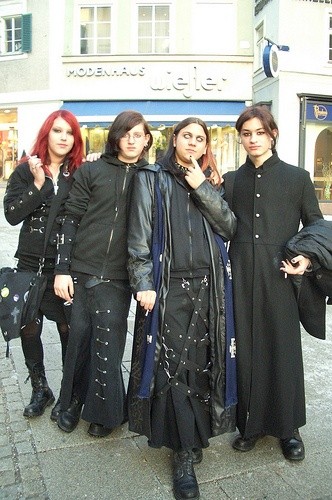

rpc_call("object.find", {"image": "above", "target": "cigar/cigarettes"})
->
[145,310,148,316]
[285,273,287,278]
[64,298,74,305]
[29,153,31,159]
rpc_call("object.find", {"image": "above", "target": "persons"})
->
[127,117,239,500]
[3,110,102,420]
[54,110,225,437]
[222,104,326,461]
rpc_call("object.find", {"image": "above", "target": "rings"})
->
[33,167,36,169]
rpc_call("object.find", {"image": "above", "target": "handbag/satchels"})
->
[0,267,48,342]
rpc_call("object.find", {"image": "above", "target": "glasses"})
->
[121,132,147,138]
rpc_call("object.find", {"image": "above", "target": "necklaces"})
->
[49,169,60,195]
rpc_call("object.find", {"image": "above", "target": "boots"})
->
[57,389,83,433]
[192,447,203,464]
[171,448,200,500]
[50,369,64,421]
[23,361,55,418]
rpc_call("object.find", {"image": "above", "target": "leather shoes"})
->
[232,430,269,452]
[88,415,128,437]
[281,428,305,461]
